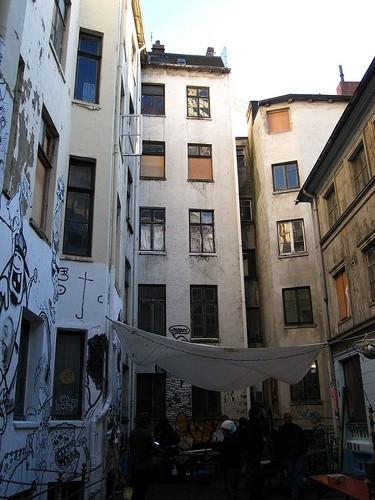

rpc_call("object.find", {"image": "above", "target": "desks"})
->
[311,474,375,500]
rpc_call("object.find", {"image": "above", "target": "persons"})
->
[211,420,242,500]
[128,414,161,500]
[236,407,270,500]
[278,413,304,500]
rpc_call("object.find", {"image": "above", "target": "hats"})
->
[221,420,235,430]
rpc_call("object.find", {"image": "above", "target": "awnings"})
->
[104,315,329,392]
